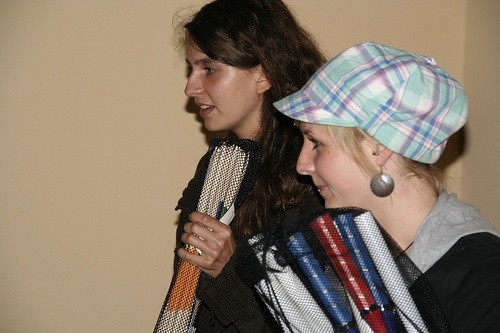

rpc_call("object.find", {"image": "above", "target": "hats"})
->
[272,37,468,166]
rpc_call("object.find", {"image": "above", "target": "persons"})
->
[153,0,360,333]
[273,40,499,333]
